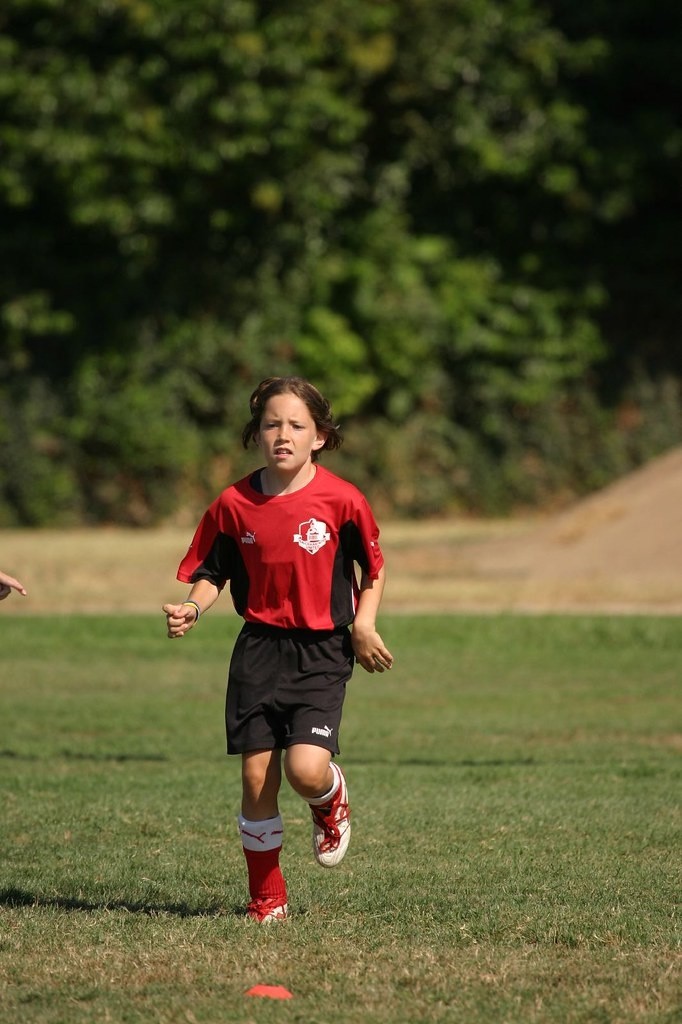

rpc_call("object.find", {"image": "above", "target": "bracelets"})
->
[181,600,200,627]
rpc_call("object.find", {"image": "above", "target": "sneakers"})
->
[246,897,288,926]
[311,762,351,868]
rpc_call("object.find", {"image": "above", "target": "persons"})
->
[161,378,393,925]
